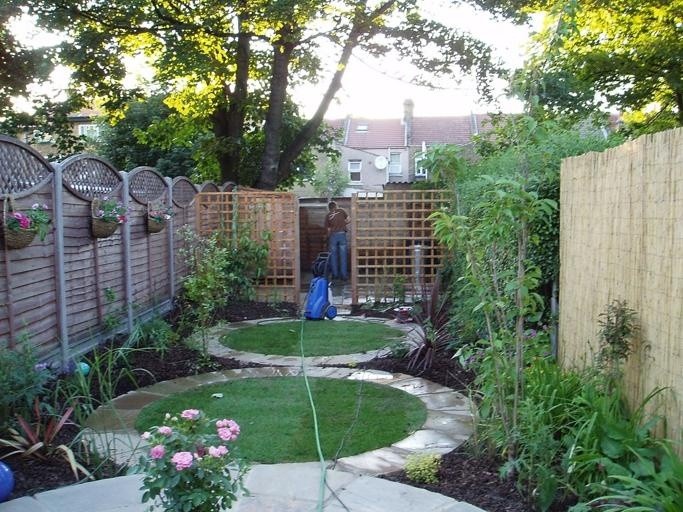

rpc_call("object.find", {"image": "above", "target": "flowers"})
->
[148,203,174,226]
[94,197,127,225]
[5,203,54,242]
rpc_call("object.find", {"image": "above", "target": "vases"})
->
[4,226,38,248]
[147,200,165,232]
[92,197,119,238]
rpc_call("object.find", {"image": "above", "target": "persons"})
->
[325,201,351,280]
